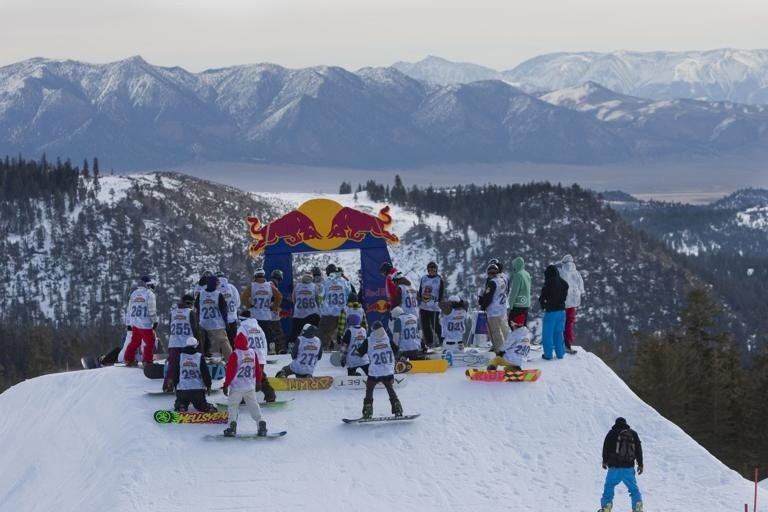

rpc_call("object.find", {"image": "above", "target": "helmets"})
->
[140,264,344,289]
[380,255,574,284]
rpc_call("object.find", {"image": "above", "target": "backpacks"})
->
[615,429,637,462]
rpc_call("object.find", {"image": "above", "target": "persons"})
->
[560,255,582,355]
[238,311,277,404]
[119,271,241,414]
[539,264,569,360]
[241,263,426,377]
[358,322,403,417]
[600,418,645,512]
[417,255,534,372]
[220,335,269,435]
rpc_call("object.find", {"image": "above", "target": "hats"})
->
[345,294,525,331]
[179,294,318,349]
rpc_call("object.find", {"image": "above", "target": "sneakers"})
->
[164,370,311,437]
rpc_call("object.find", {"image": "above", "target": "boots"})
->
[364,399,375,418]
[390,398,403,416]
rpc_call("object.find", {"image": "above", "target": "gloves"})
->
[637,466,643,475]
[602,462,607,469]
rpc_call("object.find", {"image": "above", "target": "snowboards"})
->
[215,398,293,408]
[203,431,286,443]
[341,415,420,423]
[80,355,105,369]
[143,363,224,381]
[267,376,334,390]
[153,410,228,425]
[331,346,542,388]
